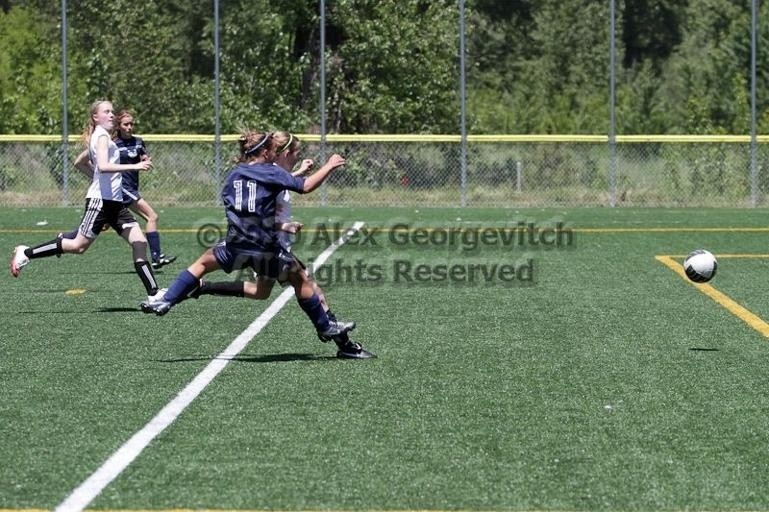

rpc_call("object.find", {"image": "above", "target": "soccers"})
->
[684,249,717,282]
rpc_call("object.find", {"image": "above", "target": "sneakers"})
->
[176,278,206,304]
[317,320,356,343]
[140,288,171,316]
[56,232,63,258]
[11,245,31,277]
[337,342,377,359]
[152,253,177,269]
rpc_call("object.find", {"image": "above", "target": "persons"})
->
[177,128,376,358]
[141,129,356,342]
[56,110,177,269]
[11,100,169,302]
